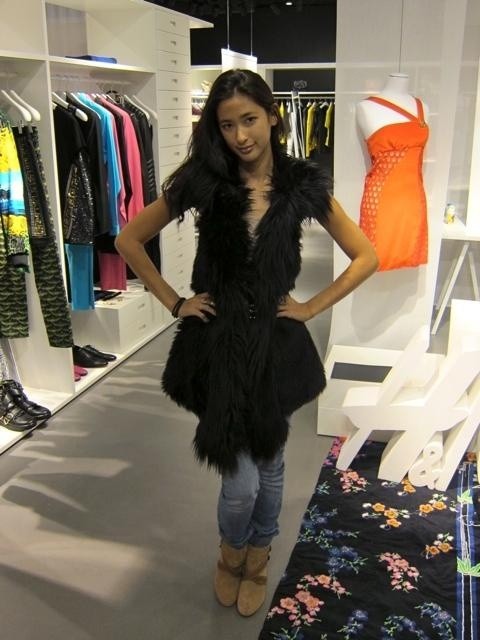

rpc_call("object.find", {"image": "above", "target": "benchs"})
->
[256,433,480,640]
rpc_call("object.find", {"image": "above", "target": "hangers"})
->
[0,70,41,134]
[52,70,159,125]
[190,98,212,107]
[274,95,334,110]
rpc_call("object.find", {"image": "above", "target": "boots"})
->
[213,539,249,608]
[237,544,272,617]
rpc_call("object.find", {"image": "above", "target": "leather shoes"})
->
[73,344,110,369]
[74,364,90,376]
[0,384,38,433]
[84,343,119,363]
[3,379,53,422]
[73,371,82,379]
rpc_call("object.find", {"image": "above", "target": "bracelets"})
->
[172,296,186,319]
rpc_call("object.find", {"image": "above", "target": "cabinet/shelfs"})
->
[155,9,197,325]
[0,0,76,455]
[42,0,161,400]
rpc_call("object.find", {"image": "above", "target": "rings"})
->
[210,300,214,306]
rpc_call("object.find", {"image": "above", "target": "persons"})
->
[355,72,427,270]
[113,69,379,618]
